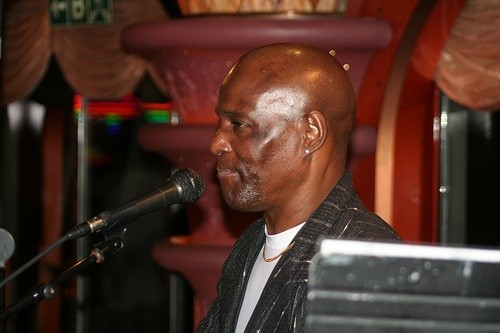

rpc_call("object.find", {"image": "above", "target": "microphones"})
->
[67,167,206,241]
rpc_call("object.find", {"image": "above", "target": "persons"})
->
[195,42,405,333]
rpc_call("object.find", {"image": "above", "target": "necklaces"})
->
[263,241,296,262]
